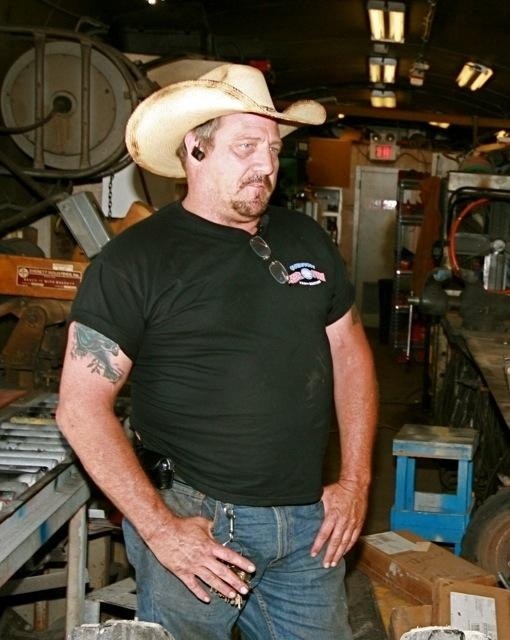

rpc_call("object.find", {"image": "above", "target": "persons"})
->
[56,63,381,640]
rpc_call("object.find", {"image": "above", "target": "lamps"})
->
[366,0,493,109]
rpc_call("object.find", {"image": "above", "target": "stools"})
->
[391,424,480,557]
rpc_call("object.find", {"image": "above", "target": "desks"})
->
[0,465,93,640]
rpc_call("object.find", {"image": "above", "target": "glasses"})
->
[249,235,290,284]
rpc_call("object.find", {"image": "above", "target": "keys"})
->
[210,505,252,609]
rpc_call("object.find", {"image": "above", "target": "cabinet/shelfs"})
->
[289,185,342,245]
[388,179,424,353]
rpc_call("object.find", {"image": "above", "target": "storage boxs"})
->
[431,580,510,640]
[369,580,412,640]
[389,605,432,640]
[354,531,498,606]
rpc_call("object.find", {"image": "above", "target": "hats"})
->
[125,63,327,178]
